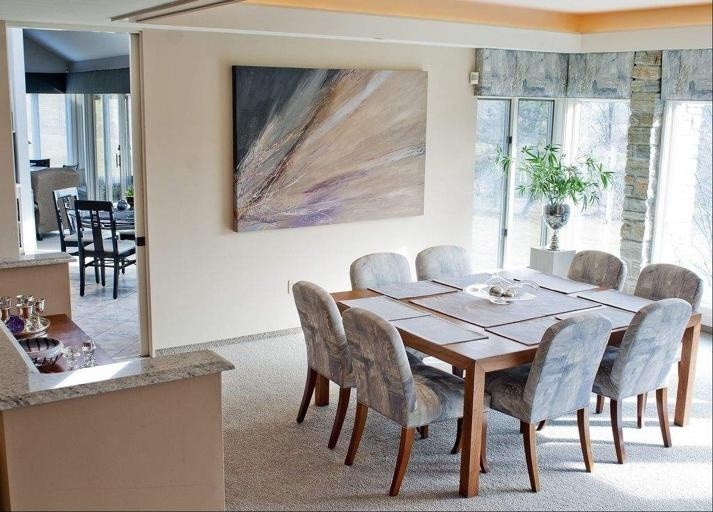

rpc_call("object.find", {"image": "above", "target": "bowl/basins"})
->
[16,337,63,371]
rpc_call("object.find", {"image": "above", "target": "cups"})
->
[61,339,96,371]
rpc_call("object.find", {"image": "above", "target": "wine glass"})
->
[0,292,46,327]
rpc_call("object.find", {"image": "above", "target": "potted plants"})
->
[495,144,617,251]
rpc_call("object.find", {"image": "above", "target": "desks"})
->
[1,314,125,373]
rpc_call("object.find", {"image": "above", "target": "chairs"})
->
[29,159,136,299]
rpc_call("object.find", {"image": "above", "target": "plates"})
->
[462,274,538,305]
